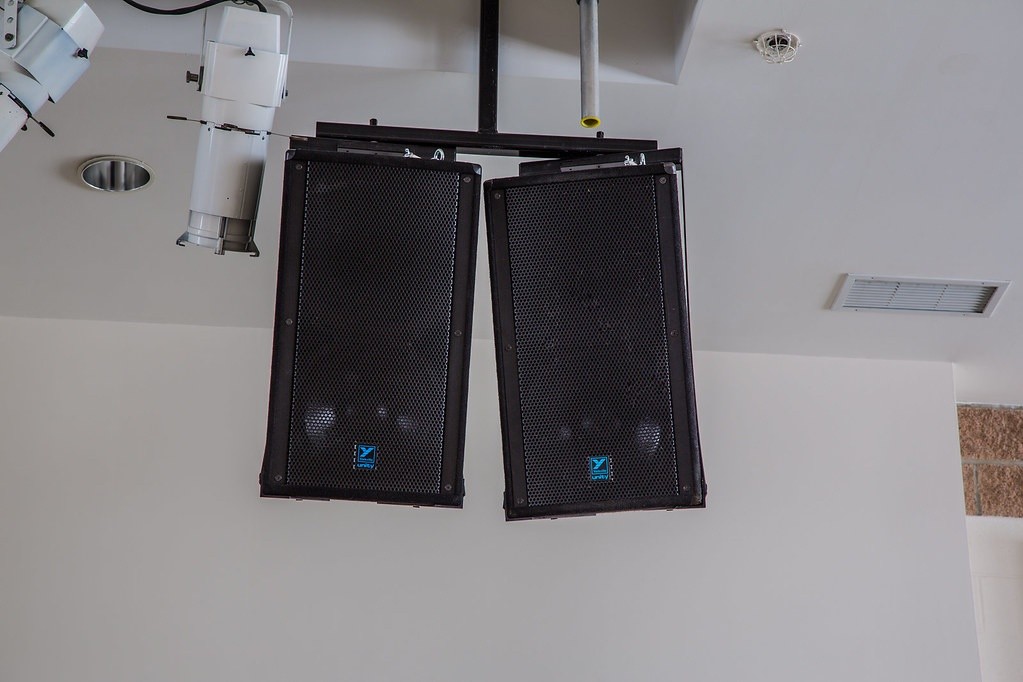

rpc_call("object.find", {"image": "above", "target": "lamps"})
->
[0,0,115,154]
[168,0,305,257]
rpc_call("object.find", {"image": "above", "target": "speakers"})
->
[482,165,707,523]
[260,150,483,503]
